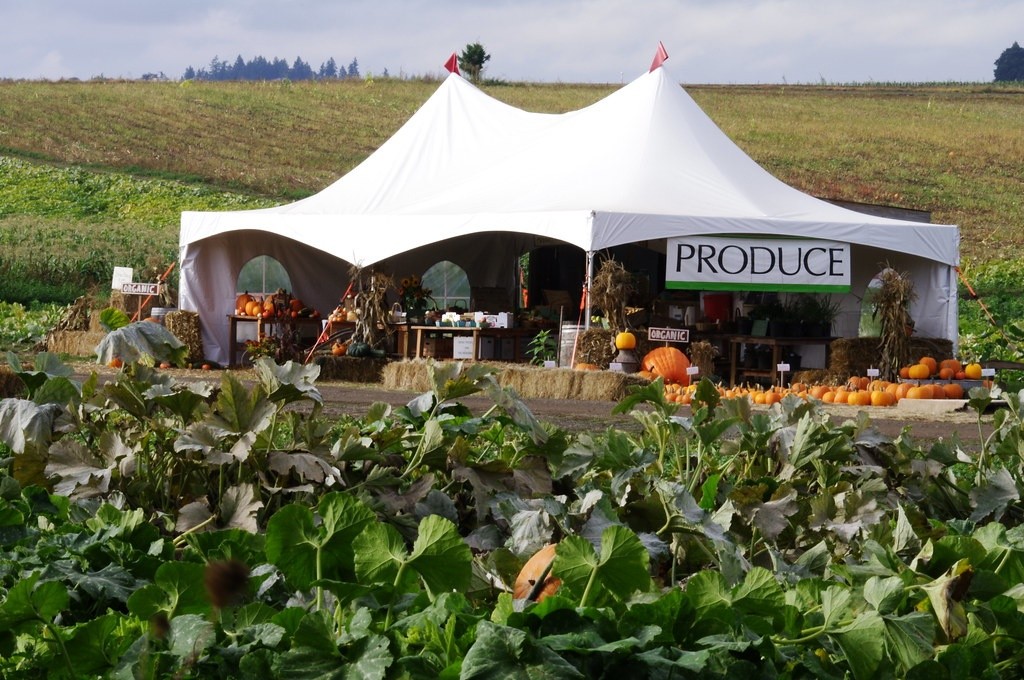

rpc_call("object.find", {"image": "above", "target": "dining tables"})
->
[328,317,421,364]
[414,325,536,362]
[731,337,839,392]
[228,313,326,374]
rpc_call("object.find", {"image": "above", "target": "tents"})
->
[178,40,960,380]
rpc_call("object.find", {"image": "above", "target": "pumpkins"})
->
[513,540,581,603]
[235,289,387,357]
[574,328,994,412]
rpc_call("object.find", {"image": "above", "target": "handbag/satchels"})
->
[388,303,407,325]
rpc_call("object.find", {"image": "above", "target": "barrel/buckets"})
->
[560,323,584,368]
